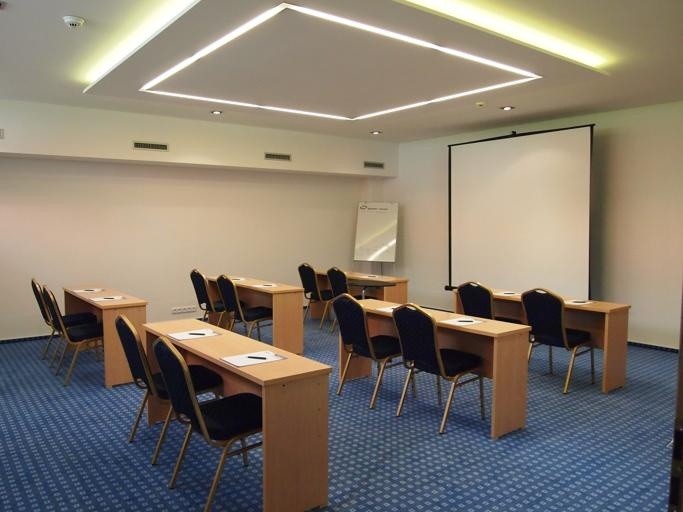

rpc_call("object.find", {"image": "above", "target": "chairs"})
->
[112,309,222,465]
[331,290,418,409]
[189,269,244,328]
[324,266,378,303]
[41,284,113,387]
[456,281,523,326]
[151,336,263,512]
[295,261,333,329]
[390,302,488,436]
[212,274,273,339]
[29,279,99,368]
[519,284,596,394]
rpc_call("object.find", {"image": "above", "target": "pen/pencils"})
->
[458,320,474,322]
[264,285,272,286]
[85,290,94,291]
[189,333,206,335]
[504,293,515,294]
[368,275,376,277]
[103,297,115,299]
[232,279,240,280]
[392,308,395,309]
[574,301,586,303]
[248,356,266,359]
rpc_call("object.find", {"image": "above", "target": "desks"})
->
[450,287,633,395]
[334,298,534,442]
[61,287,148,391]
[139,315,335,512]
[307,269,411,324]
[204,273,309,359]
